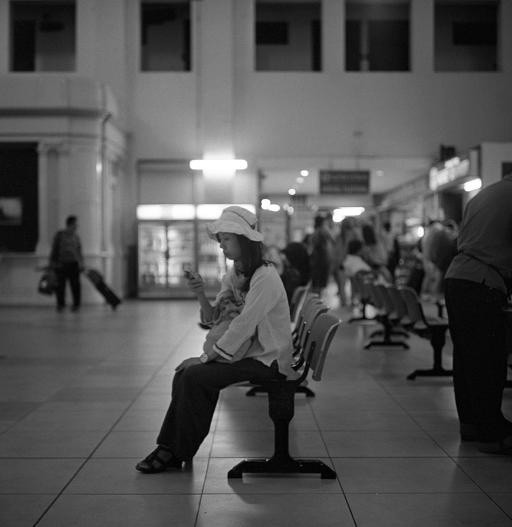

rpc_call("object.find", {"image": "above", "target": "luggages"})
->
[81,267,121,308]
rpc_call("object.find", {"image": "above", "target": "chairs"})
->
[229,278,342,481]
[347,274,453,382]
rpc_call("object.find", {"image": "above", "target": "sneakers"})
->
[477,423,512,455]
[459,419,483,442]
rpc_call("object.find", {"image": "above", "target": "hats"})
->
[206,206,265,242]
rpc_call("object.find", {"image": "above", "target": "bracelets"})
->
[199,352,210,364]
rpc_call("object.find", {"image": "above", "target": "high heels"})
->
[136,445,183,473]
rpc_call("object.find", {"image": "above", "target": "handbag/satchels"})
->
[202,294,254,365]
[37,269,58,295]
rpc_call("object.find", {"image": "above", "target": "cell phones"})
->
[184,269,196,280]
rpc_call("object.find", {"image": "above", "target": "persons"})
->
[134,204,295,474]
[265,211,458,321]
[44,215,85,312]
[443,173,511,457]
